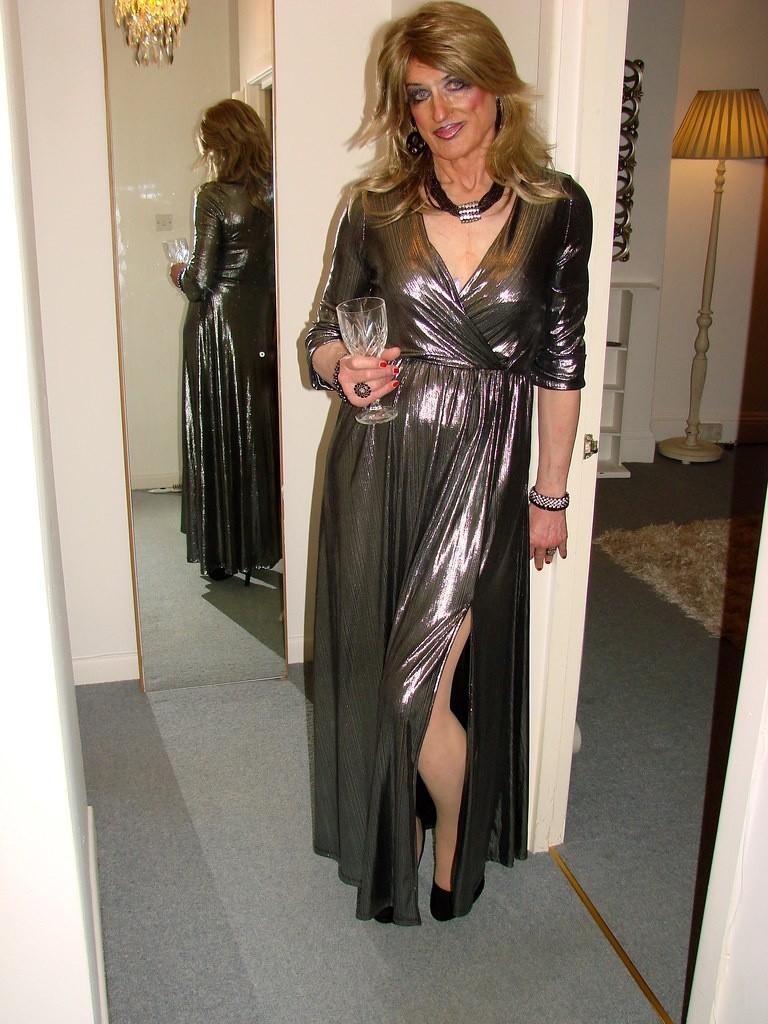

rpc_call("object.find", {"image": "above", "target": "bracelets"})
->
[333,354,351,403]
[528,486,570,510]
[176,268,185,294]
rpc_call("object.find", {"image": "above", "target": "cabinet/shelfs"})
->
[596,283,661,481]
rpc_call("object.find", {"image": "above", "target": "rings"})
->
[546,547,558,556]
[354,382,371,399]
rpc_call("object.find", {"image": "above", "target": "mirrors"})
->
[99,0,290,691]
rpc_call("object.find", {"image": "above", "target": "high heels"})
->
[207,566,250,586]
[430,826,462,921]
[374,815,425,923]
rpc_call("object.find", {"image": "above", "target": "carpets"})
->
[594,513,730,640]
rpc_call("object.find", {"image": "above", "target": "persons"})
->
[305,0,593,924]
[168,98,283,587]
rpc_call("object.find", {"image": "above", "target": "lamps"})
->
[657,90,768,466]
[114,0,192,69]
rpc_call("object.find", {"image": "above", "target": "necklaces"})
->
[424,150,507,224]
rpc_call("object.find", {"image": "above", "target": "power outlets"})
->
[697,423,722,442]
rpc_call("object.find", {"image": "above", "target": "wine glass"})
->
[162,237,190,295]
[336,297,399,425]
